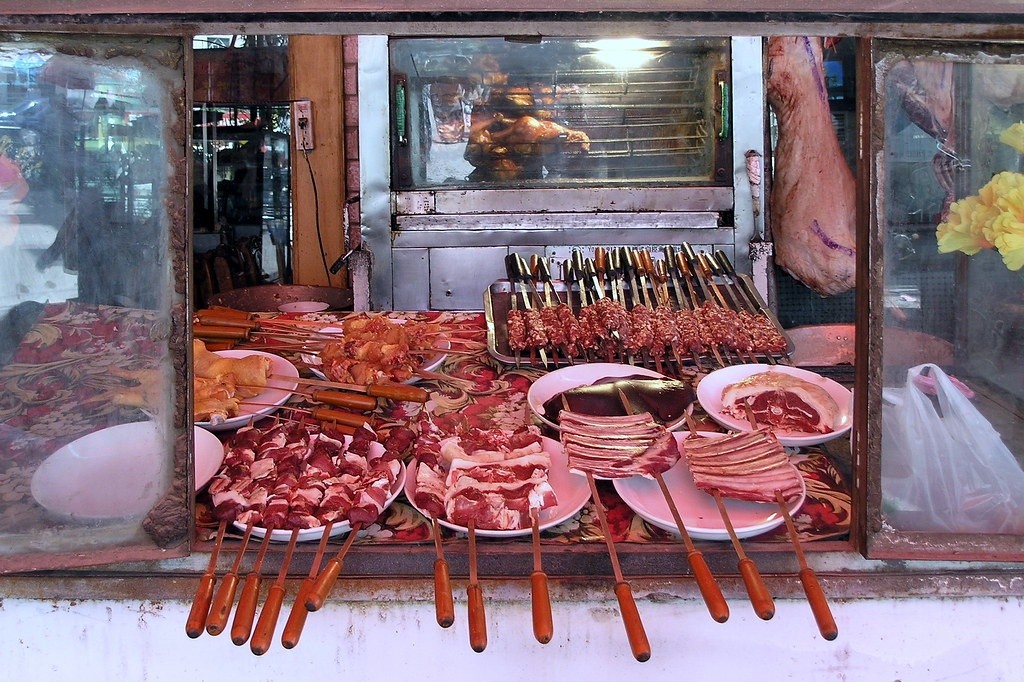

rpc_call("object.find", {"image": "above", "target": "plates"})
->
[211,434,406,542]
[300,320,451,385]
[612,431,806,540]
[526,363,694,432]
[402,435,595,537]
[136,349,299,430]
[30,421,224,522]
[697,364,854,447]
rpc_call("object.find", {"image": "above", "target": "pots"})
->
[207,284,353,312]
[777,323,955,368]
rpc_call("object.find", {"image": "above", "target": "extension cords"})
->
[294,100,315,150]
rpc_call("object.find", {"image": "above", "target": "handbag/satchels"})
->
[880,359,1023,534]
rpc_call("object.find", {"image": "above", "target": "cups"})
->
[278,301,330,312]
